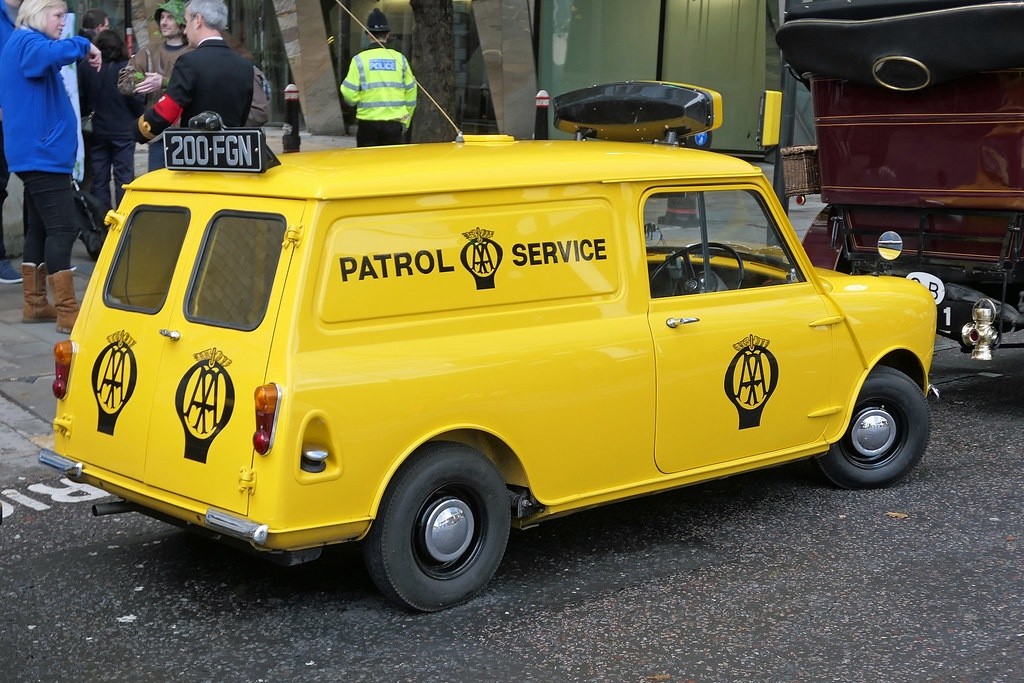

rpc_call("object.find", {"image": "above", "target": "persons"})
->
[77,0,272,227]
[0,0,25,284]
[340,8,417,147]
[0,0,102,335]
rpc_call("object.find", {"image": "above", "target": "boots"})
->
[47,270,79,333]
[21,262,57,323]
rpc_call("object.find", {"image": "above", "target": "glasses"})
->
[102,25,111,29]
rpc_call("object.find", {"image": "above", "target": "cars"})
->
[36,79,941,611]
[775,0,1024,360]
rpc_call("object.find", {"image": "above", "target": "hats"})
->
[153,0,187,26]
[365,8,391,31]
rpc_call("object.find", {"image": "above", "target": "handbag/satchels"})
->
[73,179,110,262]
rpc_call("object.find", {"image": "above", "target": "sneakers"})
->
[0,259,23,283]
